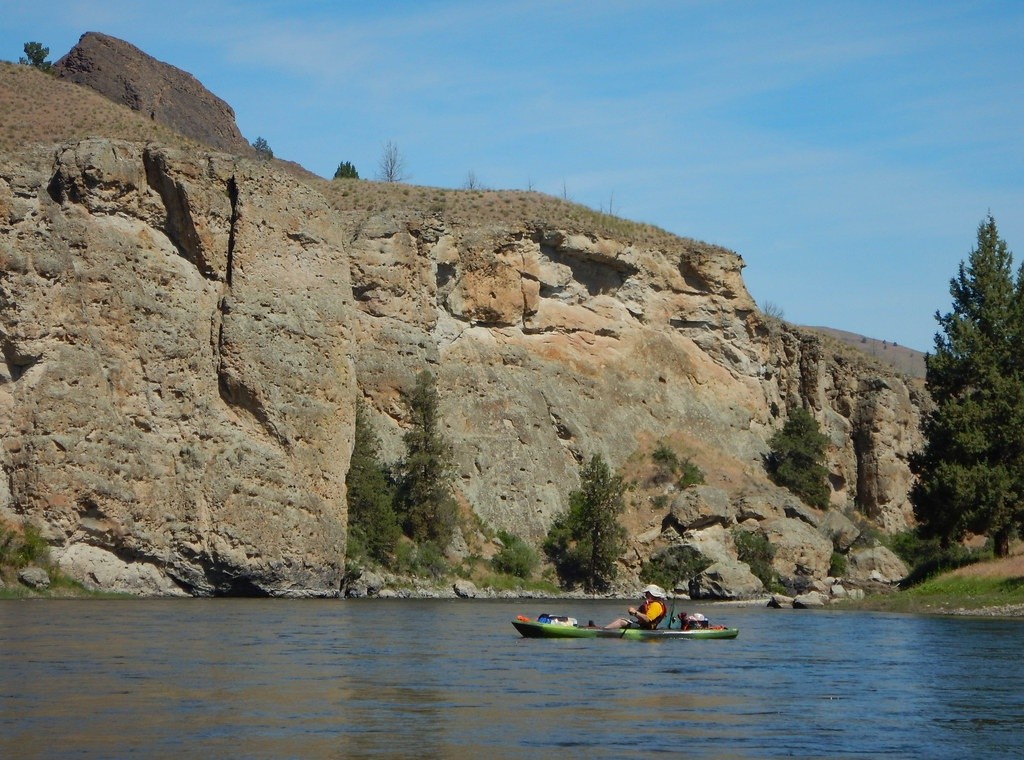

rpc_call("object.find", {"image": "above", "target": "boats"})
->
[511,611,741,640]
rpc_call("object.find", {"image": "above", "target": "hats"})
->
[643,585,666,597]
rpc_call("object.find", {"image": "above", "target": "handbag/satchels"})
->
[538,613,577,627]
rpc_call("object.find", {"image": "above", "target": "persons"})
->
[605,584,666,629]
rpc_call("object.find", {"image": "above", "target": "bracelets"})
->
[635,612,638,615]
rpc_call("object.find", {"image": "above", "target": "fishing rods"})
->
[667,557,683,630]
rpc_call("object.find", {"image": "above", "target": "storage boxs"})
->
[681,620,709,630]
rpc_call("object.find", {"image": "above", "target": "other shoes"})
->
[589,620,596,627]
